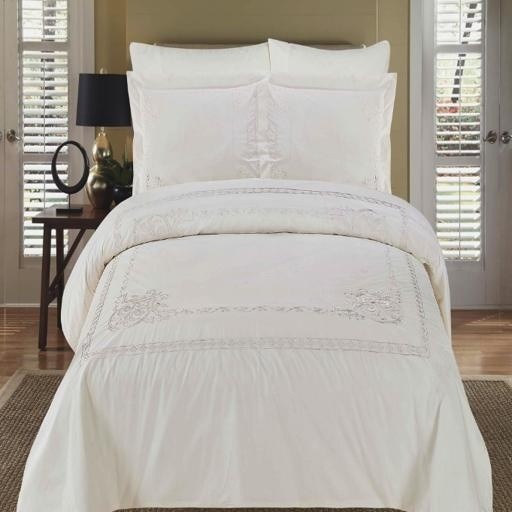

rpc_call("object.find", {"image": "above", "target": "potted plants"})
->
[109,154,134,205]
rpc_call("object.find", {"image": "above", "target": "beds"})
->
[27,45,489,510]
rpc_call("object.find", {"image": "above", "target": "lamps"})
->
[76,74,131,209]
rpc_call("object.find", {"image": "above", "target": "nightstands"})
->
[32,200,103,348]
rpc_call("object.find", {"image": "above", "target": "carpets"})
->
[0,365,512,512]
